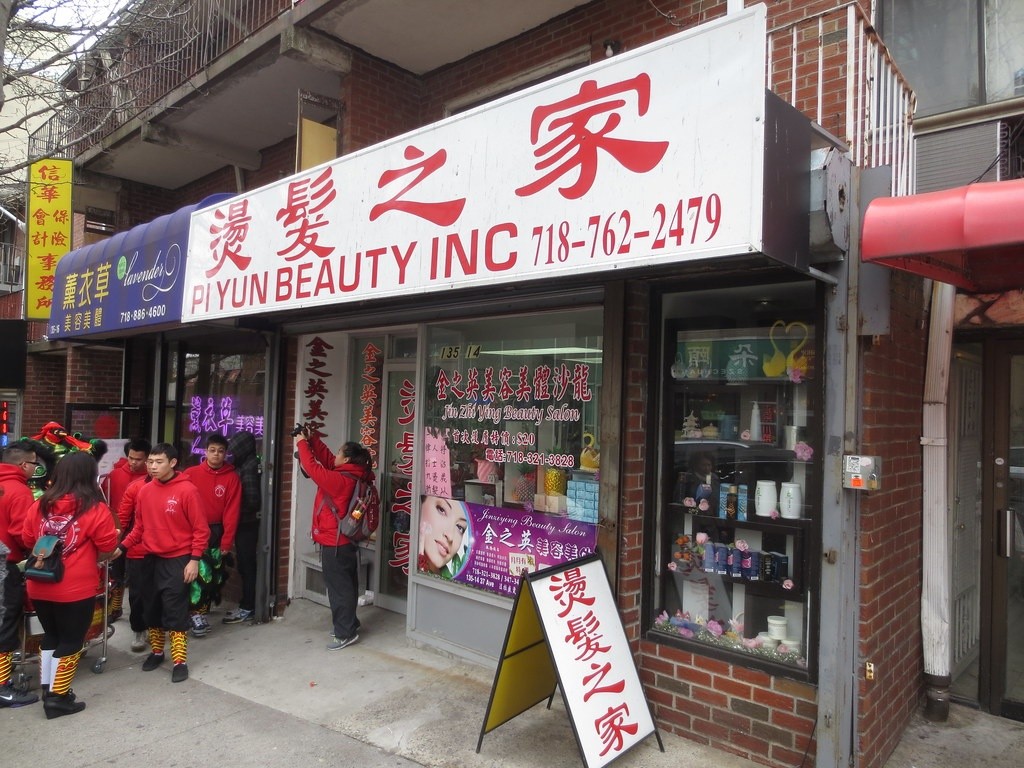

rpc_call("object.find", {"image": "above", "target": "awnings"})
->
[860,178,1024,292]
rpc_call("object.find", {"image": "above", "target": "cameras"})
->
[290,423,308,438]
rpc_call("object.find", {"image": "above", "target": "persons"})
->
[297,425,376,651]
[688,451,719,501]
[0,431,257,720]
[418,495,467,579]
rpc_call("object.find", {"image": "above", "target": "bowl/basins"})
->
[703,423,719,437]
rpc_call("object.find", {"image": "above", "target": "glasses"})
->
[19,461,39,466]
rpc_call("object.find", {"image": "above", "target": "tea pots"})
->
[670,352,712,379]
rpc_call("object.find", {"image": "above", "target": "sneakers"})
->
[222,608,254,624]
[202,616,212,633]
[0,679,38,709]
[327,633,359,650]
[189,615,207,637]
[329,620,360,637]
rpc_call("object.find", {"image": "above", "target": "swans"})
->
[762,319,810,378]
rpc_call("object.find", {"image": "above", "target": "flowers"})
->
[783,580,794,589]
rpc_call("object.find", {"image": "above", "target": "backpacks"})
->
[23,494,95,583]
[325,472,380,542]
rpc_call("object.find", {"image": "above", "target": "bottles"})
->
[727,485,737,520]
[780,482,801,519]
[755,480,777,516]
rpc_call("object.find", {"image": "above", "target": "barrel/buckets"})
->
[720,416,740,440]
[782,425,803,450]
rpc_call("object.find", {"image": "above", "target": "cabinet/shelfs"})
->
[660,377,811,652]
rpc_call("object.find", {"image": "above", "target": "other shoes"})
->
[43,699,86,720]
[10,649,40,662]
[142,652,165,671]
[130,630,147,653]
[40,684,76,702]
[108,608,122,624]
[172,662,188,682]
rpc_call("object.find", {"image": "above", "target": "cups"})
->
[759,616,801,653]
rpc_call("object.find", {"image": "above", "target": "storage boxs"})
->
[703,544,760,581]
